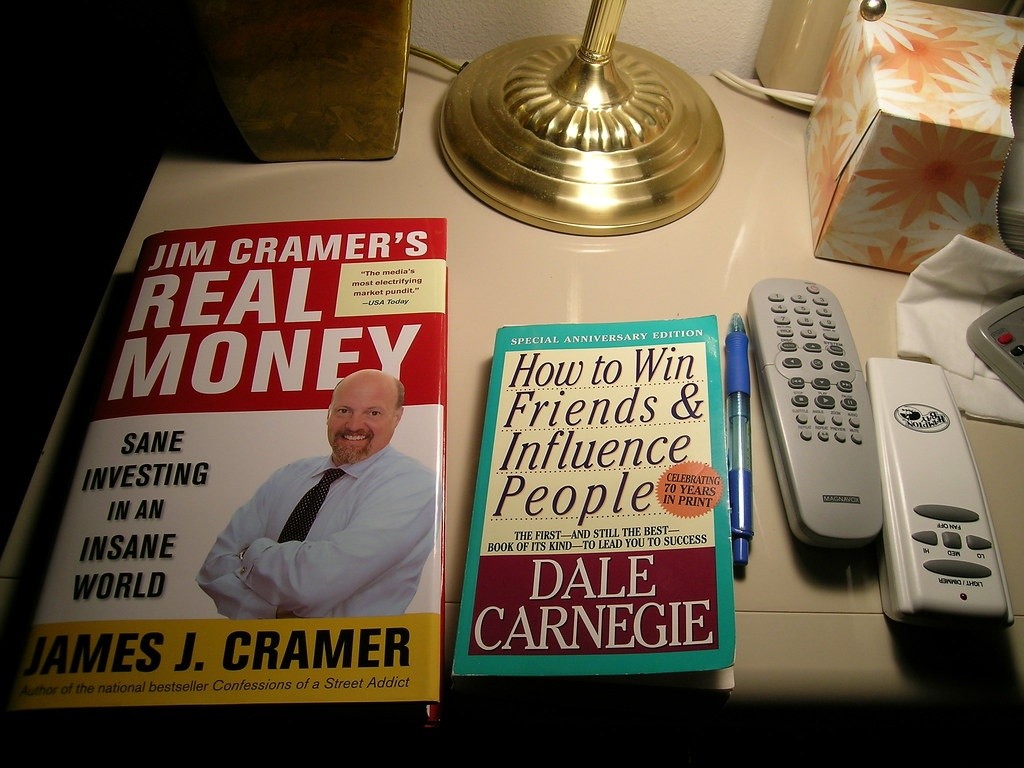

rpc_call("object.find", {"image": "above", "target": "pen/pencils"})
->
[725,313,757,567]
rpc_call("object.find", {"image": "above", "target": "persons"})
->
[197,370,436,620]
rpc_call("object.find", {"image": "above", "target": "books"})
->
[7,217,449,712]
[452,312,737,694]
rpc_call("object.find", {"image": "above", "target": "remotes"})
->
[866,357,1015,633]
[966,295,1024,402]
[746,279,883,549]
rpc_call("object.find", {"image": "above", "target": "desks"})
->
[0,55,1024,712]
